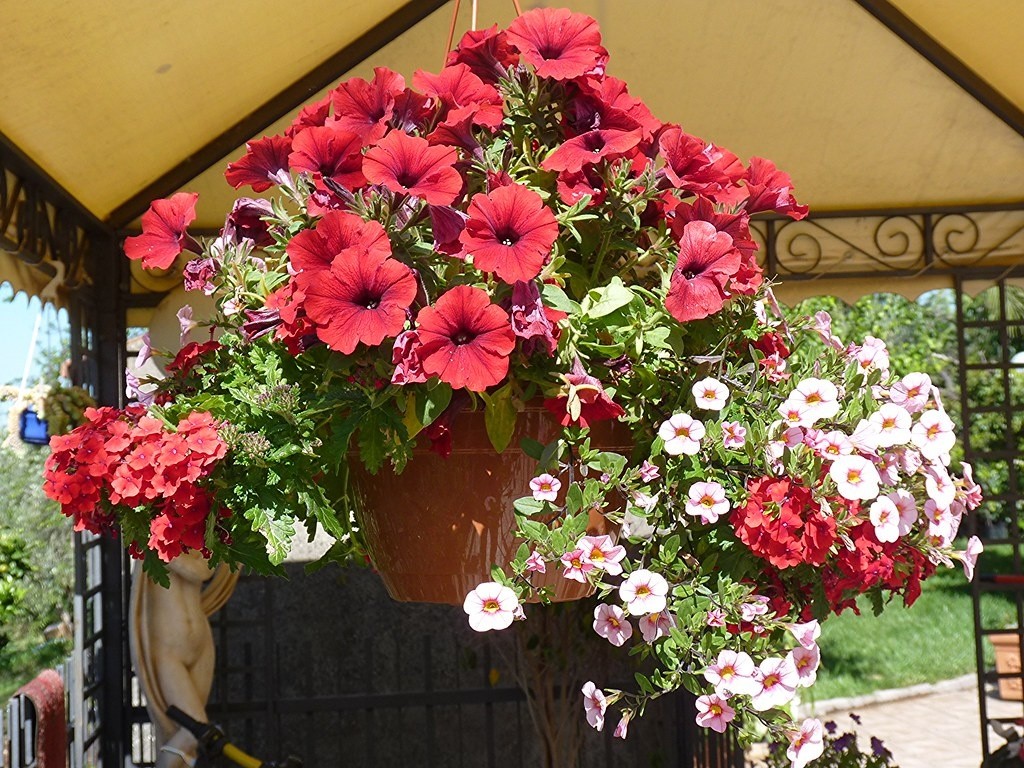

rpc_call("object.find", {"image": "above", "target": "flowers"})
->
[45,4,983,768]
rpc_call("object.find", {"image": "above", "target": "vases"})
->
[350,393,634,605]
